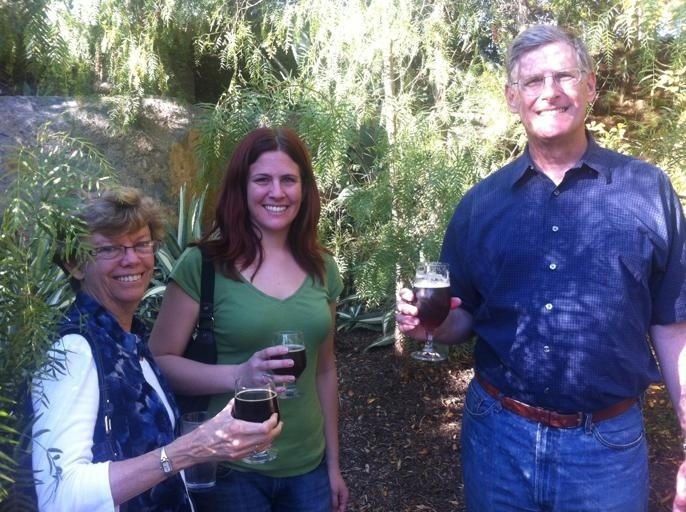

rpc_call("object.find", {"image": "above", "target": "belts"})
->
[476,372,639,428]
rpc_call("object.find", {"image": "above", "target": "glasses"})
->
[511,68,585,91]
[88,242,162,259]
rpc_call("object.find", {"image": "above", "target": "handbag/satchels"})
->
[148,243,217,416]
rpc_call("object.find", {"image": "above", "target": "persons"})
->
[146,129,349,512]
[15,187,284,512]
[397,27,686,512]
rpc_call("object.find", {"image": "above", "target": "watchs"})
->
[160,445,175,478]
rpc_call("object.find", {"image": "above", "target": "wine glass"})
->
[274,330,308,399]
[414,264,451,361]
[232,375,279,464]
